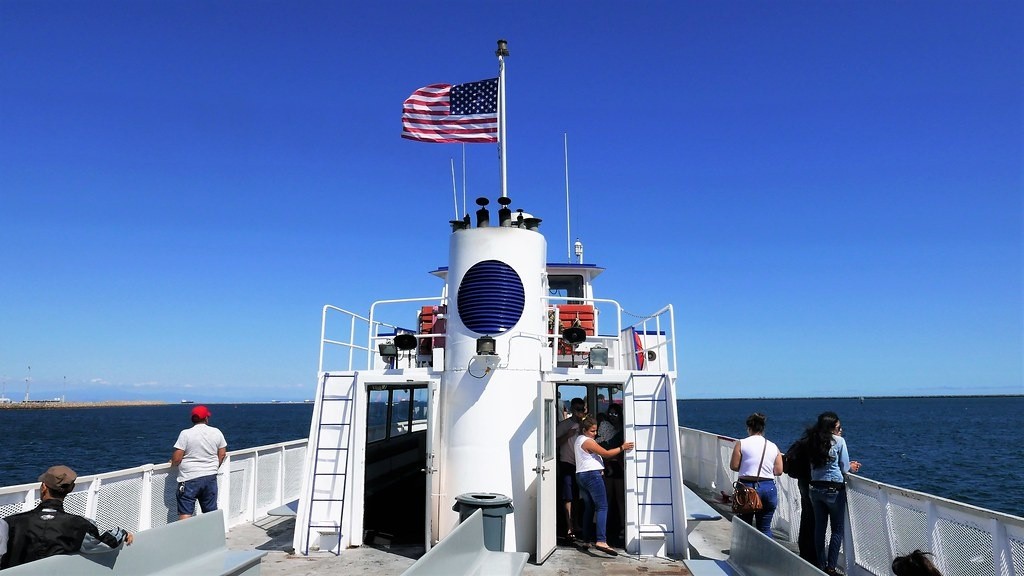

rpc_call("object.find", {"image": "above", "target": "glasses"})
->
[837,425,841,432]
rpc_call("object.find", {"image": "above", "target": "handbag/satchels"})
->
[732,481,763,514]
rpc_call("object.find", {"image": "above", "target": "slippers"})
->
[582,542,595,549]
[595,542,618,557]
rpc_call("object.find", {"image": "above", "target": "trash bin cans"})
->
[452,493,515,551]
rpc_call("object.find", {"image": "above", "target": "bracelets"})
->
[620,446,623,451]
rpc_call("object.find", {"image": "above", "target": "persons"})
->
[574,416,634,556]
[730,413,784,538]
[796,412,862,576]
[0,465,132,569]
[556,391,623,539]
[892,549,943,576]
[171,405,227,520]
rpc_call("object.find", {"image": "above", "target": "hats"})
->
[191,405,211,420]
[37,465,77,491]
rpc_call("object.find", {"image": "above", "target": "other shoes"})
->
[824,566,848,576]
[565,528,578,541]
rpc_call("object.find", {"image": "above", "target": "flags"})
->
[401,77,499,143]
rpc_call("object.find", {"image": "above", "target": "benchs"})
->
[0,509,269,576]
[683,484,723,535]
[268,499,299,517]
[682,515,829,576]
[400,509,531,576]
